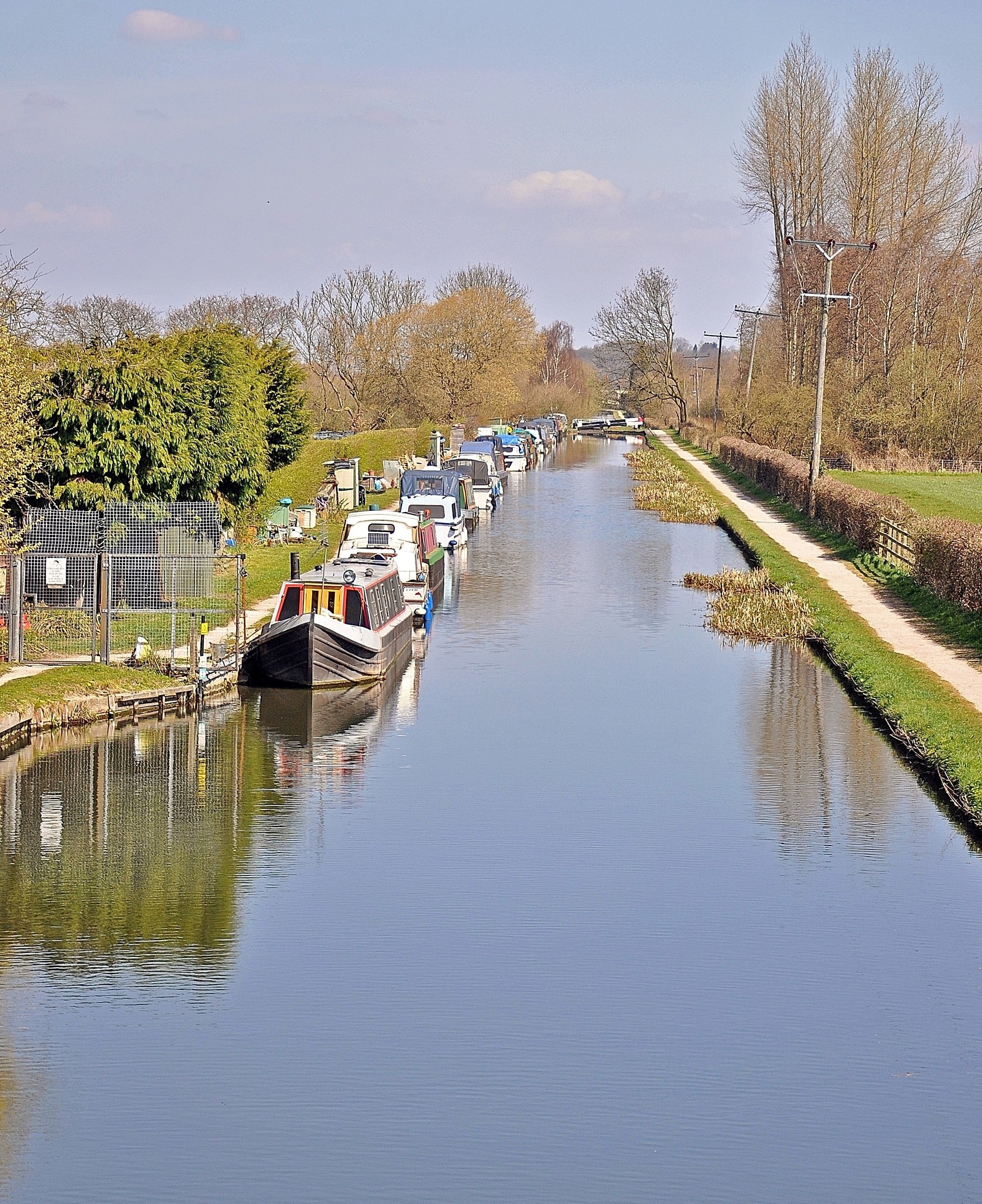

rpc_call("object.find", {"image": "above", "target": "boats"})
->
[402,466,480,529]
[399,494,468,550]
[460,436,508,485]
[339,505,444,616]
[244,539,414,689]
[494,413,567,471]
[443,452,504,509]
